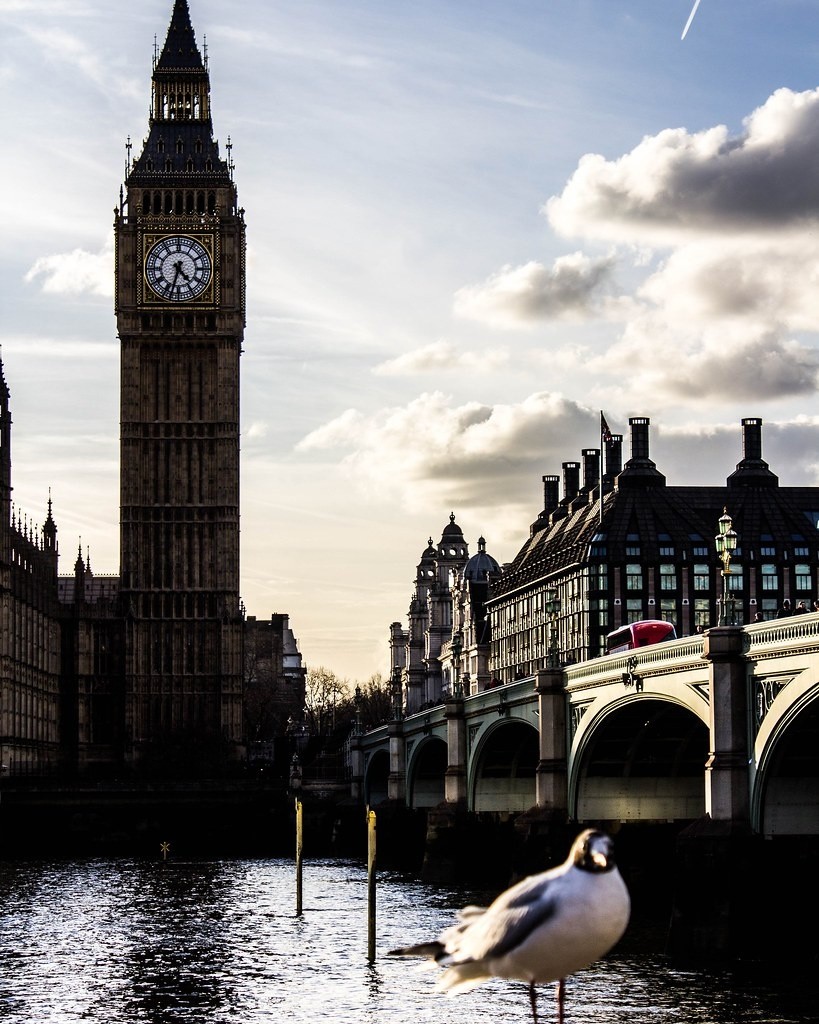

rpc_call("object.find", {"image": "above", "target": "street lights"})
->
[353,684,363,735]
[713,504,738,628]
[447,631,466,700]
[388,662,403,719]
[543,581,562,667]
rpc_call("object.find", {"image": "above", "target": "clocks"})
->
[143,233,214,305]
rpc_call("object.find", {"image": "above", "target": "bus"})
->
[604,619,677,656]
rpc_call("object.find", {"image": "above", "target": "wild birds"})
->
[384,827,632,1024]
